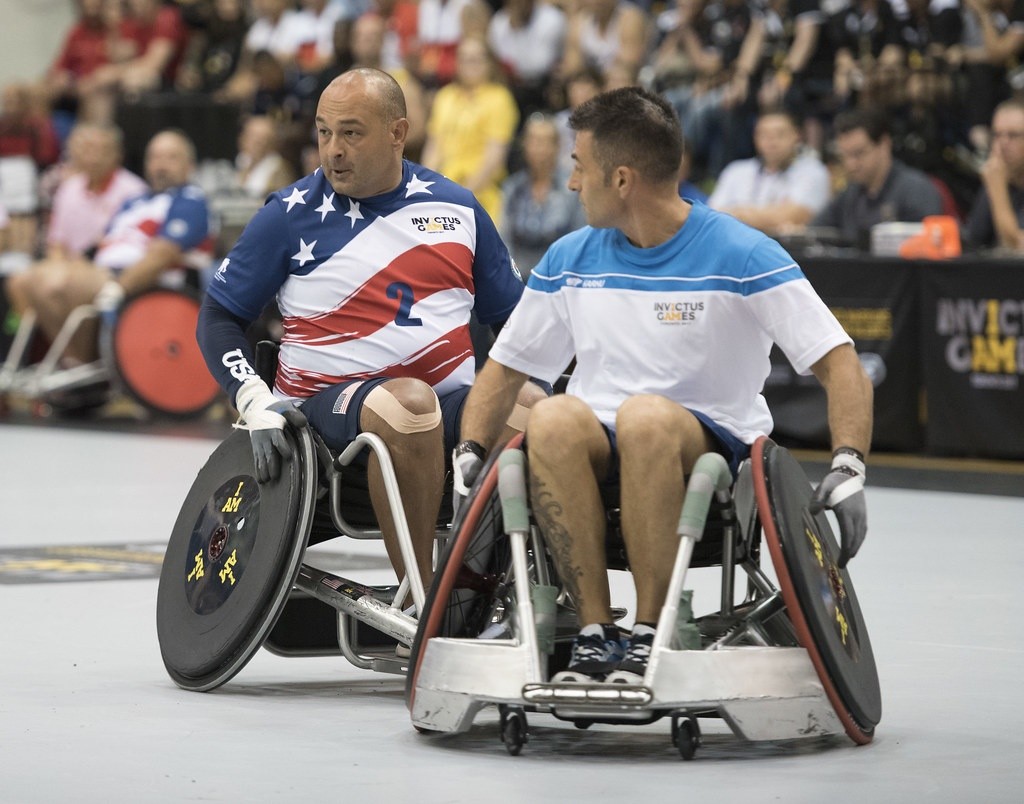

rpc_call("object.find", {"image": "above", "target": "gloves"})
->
[449,440,487,524]
[809,447,867,568]
[235,379,308,484]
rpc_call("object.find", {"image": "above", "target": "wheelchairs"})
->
[0,260,224,423]
[397,352,885,763]
[154,337,553,695]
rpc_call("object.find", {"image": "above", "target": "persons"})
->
[196,66,554,658]
[452,88,874,689]
[1,0,1024,366]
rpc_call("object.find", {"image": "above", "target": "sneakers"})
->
[607,620,657,685]
[554,622,624,689]
